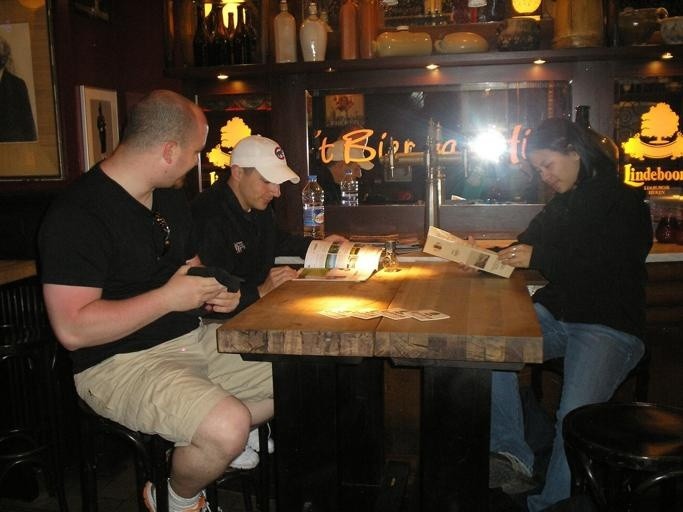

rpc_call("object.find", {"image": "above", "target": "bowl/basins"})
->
[661,15,682,44]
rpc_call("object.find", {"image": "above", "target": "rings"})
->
[511,254,515,258]
[512,247,516,252]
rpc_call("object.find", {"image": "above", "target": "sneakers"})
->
[488,451,536,496]
[143,479,222,512]
[229,423,274,470]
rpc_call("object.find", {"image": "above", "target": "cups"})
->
[433,31,489,56]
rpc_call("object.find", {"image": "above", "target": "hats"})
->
[231,134,302,185]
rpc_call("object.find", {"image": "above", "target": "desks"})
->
[216,242,546,511]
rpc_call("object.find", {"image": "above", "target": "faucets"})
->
[423,144,437,178]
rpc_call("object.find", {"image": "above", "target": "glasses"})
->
[152,210,172,262]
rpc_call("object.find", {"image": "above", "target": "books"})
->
[423,225,512,279]
[290,238,382,282]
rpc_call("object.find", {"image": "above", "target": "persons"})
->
[453,114,654,510]
[33,85,275,512]
[0,37,35,142]
[305,138,375,203]
[181,132,391,492]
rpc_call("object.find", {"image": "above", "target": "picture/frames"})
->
[78,86,121,179]
[0,0,68,184]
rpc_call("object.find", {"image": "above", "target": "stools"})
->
[560,396,682,511]
[0,425,59,511]
[525,332,653,402]
[77,398,270,512]
[0,259,52,496]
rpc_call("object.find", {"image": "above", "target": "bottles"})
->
[358,0,382,58]
[340,168,359,207]
[370,31,432,59]
[338,0,358,60]
[382,240,400,272]
[299,1,328,62]
[494,18,542,52]
[653,216,683,246]
[194,0,256,64]
[274,0,297,63]
[301,175,326,239]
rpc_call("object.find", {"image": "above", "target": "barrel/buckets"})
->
[542,0,605,49]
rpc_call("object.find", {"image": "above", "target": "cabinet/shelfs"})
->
[644,263,683,403]
